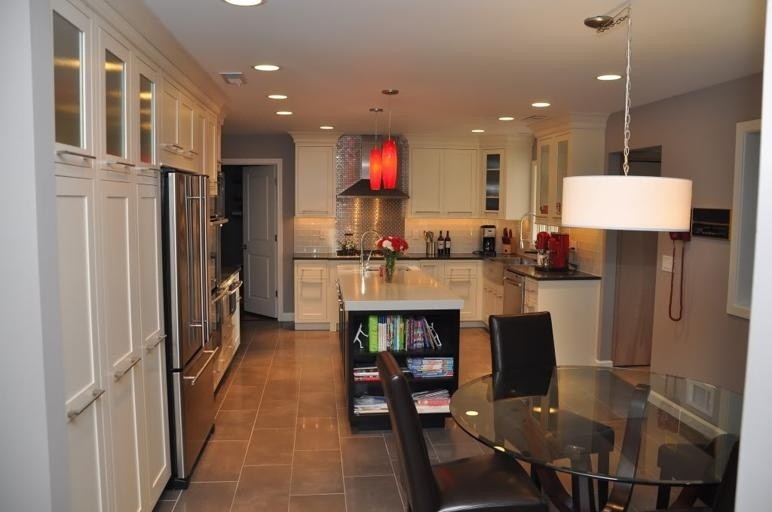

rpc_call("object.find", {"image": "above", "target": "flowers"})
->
[376,233,410,257]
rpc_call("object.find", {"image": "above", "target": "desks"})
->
[446,366,743,511]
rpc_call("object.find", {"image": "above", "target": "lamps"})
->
[380,88,404,191]
[554,3,696,236]
[366,106,386,192]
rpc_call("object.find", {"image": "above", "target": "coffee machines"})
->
[480,225,496,256]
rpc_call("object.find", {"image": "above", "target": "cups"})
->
[502,243,511,254]
[426,241,436,255]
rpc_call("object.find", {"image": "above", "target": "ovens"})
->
[212,273,245,350]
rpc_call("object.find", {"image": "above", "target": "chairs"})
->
[488,309,616,511]
[372,350,551,512]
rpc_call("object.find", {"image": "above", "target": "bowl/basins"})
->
[539,205,548,214]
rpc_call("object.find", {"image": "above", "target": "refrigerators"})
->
[164,168,219,487]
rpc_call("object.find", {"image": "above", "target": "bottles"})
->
[445,230,451,255]
[438,231,444,255]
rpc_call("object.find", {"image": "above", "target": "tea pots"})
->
[336,234,356,254]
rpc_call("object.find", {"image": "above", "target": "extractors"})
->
[336,134,413,200]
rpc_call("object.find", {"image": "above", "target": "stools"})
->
[653,430,739,510]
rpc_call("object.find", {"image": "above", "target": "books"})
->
[350,310,454,414]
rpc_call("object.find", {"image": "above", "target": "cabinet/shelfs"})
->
[533,128,606,226]
[129,178,181,512]
[420,258,485,327]
[524,278,598,366]
[481,277,505,327]
[158,74,223,179]
[477,145,529,221]
[295,259,362,332]
[124,53,161,173]
[347,311,459,429]
[57,172,145,512]
[409,146,473,219]
[294,142,338,217]
[53,0,131,169]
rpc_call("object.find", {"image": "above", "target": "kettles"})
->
[549,232,569,271]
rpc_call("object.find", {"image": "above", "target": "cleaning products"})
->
[502,227,515,255]
[568,246,578,271]
[423,229,437,256]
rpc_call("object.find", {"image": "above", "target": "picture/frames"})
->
[724,116,762,320]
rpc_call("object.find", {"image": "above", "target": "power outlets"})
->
[570,241,578,254]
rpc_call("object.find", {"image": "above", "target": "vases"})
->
[383,254,397,283]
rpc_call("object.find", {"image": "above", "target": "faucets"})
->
[518,212,536,241]
[359,229,382,254]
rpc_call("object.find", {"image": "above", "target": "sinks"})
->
[524,249,539,255]
[366,264,419,273]
